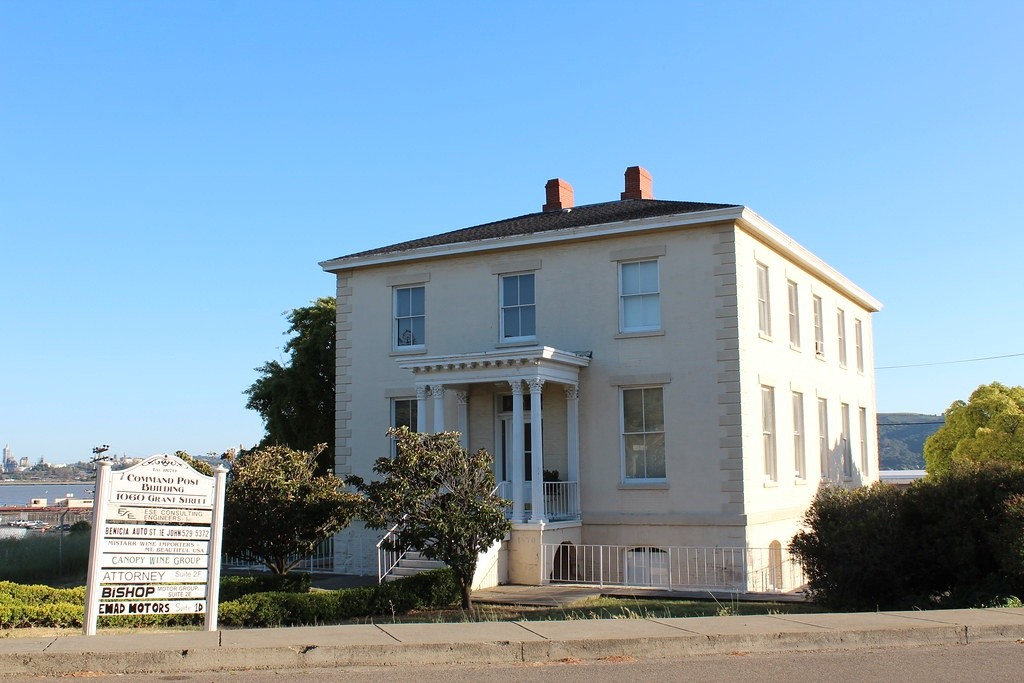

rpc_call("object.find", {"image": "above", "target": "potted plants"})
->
[543,469,566,515]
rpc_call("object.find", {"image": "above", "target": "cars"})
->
[7,518,74,532]
